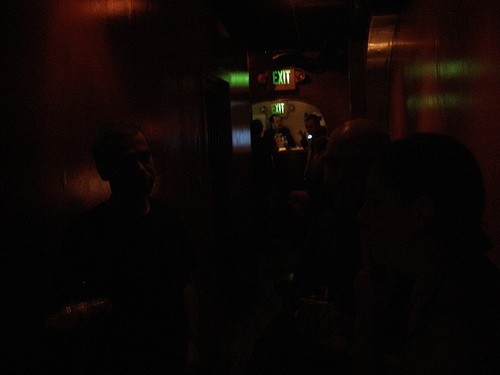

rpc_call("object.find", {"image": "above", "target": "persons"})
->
[63,124,199,375]
[294,132,499,374]
[262,114,295,148]
[250,118,271,158]
[291,117,390,285]
[298,112,329,181]
